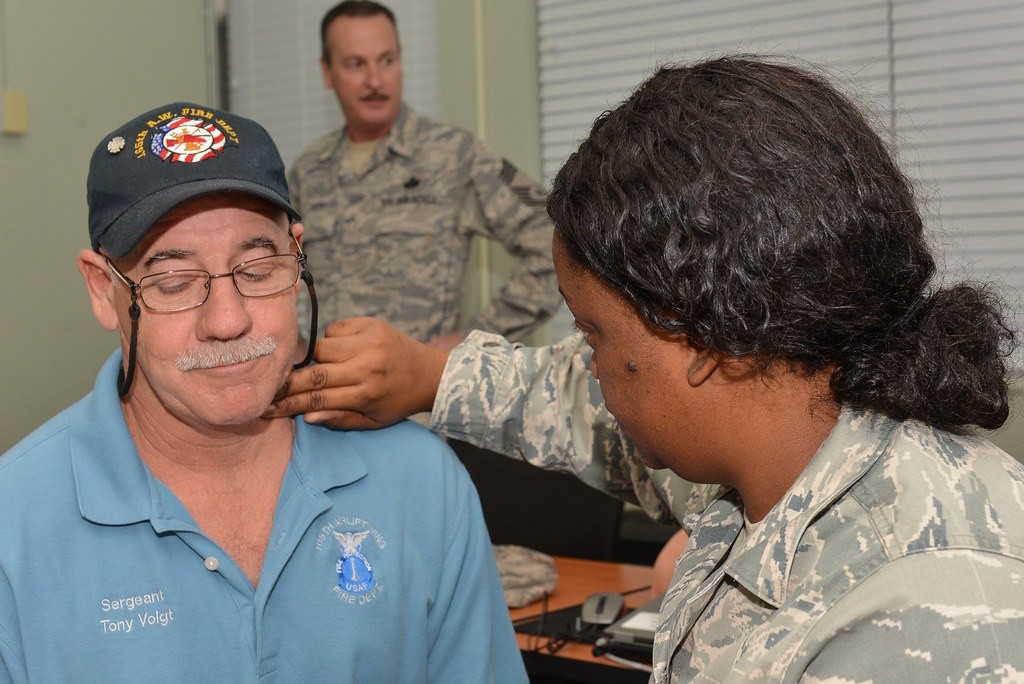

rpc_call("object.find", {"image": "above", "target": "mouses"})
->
[582,593,626,628]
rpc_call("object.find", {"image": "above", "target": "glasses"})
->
[96,227,308,312]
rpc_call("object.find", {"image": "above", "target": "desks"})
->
[508,557,652,675]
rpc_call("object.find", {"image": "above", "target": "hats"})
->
[87,102,302,257]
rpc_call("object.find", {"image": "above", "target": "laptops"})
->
[602,592,665,654]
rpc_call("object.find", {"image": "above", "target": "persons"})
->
[260,56,1023,684]
[646,523,692,603]
[0,99,528,683]
[279,1,565,366]
